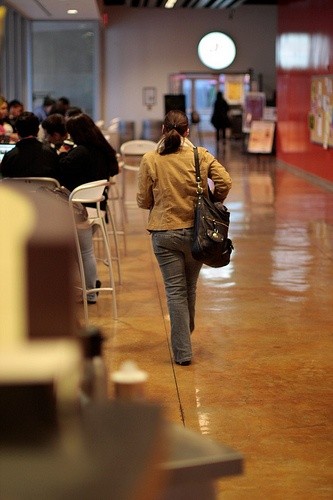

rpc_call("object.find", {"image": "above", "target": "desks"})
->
[0,404,244,500]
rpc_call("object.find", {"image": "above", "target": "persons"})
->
[189,112,203,147]
[210,90,231,153]
[136,111,232,366]
[0,94,120,304]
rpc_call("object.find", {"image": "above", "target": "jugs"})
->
[82,325,107,399]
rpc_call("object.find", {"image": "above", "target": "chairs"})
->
[3,140,157,322]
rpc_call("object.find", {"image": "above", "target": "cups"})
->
[110,371,150,399]
[63,140,74,151]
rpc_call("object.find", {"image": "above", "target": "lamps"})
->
[199,32,236,70]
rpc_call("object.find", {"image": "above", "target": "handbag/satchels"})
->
[191,185,234,268]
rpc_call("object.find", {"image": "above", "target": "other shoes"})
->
[175,360,191,366]
[96,280,102,297]
[223,137,226,139]
[217,138,220,141]
[87,300,97,305]
